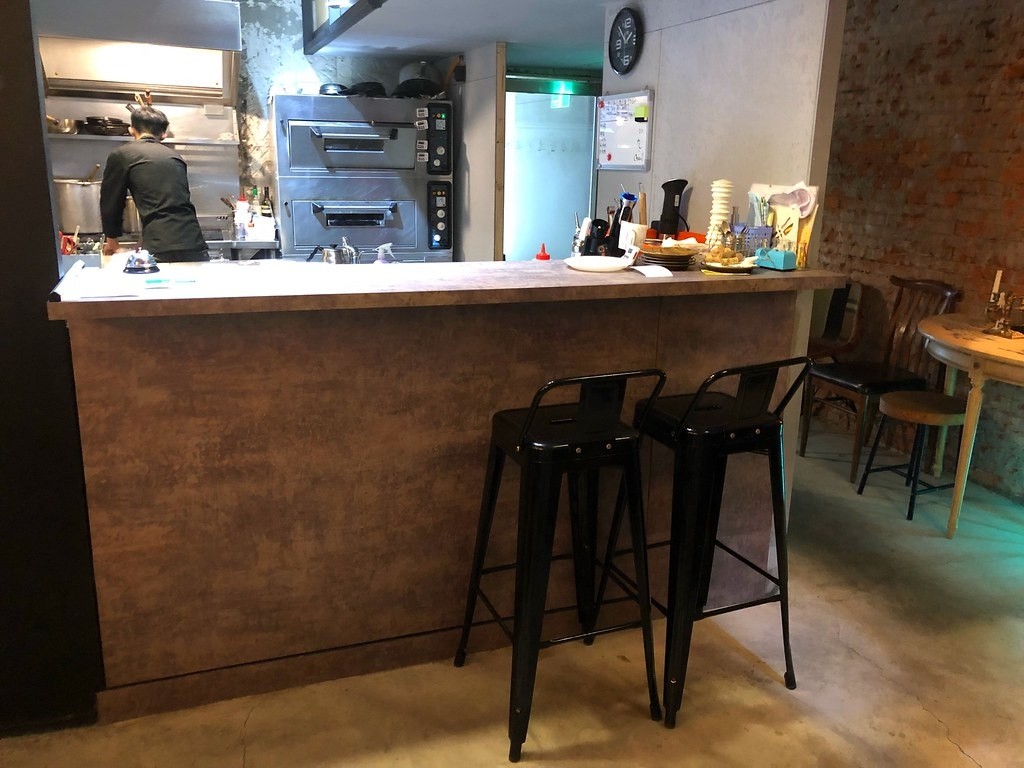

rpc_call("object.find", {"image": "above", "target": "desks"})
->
[917,313,1024,541]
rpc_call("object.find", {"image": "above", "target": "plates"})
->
[565,256,628,272]
[641,247,698,270]
[702,262,759,272]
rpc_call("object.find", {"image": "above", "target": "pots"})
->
[53,179,104,234]
[85,117,129,135]
[398,61,441,96]
[46,115,84,134]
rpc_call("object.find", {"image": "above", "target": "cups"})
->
[618,221,648,252]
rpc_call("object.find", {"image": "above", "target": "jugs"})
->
[306,237,364,264]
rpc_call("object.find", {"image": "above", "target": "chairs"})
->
[798,272,965,484]
[799,280,865,418]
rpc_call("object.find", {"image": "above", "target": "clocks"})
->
[608,6,644,75]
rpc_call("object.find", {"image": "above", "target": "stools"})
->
[586,354,815,728]
[857,390,968,521]
[454,368,670,762]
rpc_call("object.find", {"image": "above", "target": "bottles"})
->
[235,193,249,225]
[261,187,271,217]
[249,185,261,217]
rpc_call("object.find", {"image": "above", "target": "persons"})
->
[99,107,211,263]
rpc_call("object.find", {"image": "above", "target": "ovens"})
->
[271,95,454,263]
